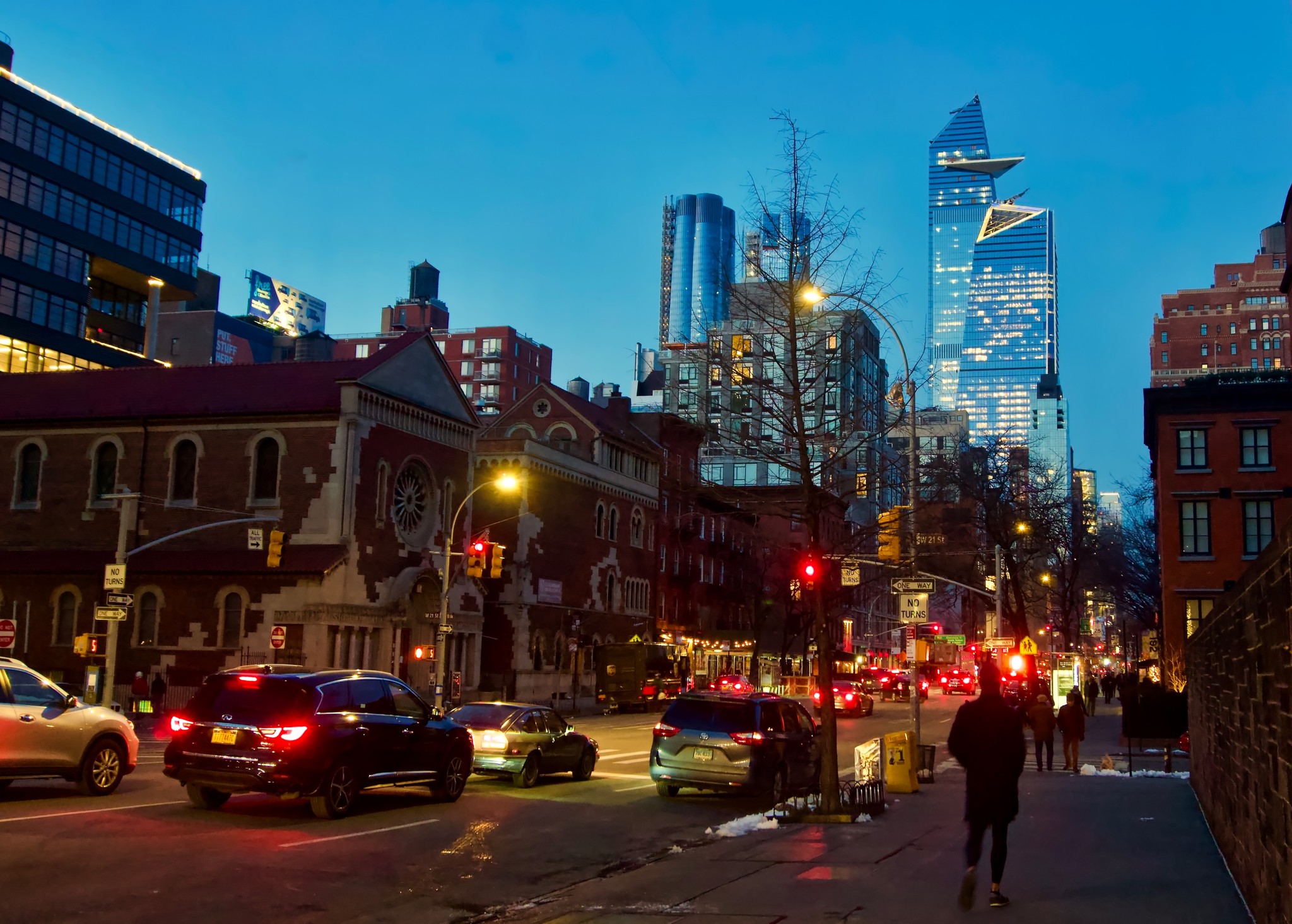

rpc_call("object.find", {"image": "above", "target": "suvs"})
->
[162,664,476,820]
[0,656,140,796]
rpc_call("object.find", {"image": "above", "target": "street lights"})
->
[435,474,519,718]
[800,286,921,781]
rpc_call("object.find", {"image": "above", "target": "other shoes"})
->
[1104,702,1107,704]
[1108,702,1111,704]
[1047,767,1053,771]
[151,714,158,718]
[1063,764,1072,770]
[134,713,140,719]
[1037,766,1043,771]
[1073,768,1079,773]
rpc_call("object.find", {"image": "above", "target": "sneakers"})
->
[989,889,1010,907]
[957,871,976,911]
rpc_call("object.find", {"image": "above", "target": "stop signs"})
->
[269,626,286,649]
[0,619,17,648]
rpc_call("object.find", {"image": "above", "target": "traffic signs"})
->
[1094,651,1103,655]
[906,625,916,660]
[248,528,264,550]
[106,593,134,606]
[95,607,127,620]
[438,623,454,634]
[1079,618,1092,631]
[863,633,873,637]
[891,579,935,594]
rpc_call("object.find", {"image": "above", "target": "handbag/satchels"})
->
[128,694,135,699]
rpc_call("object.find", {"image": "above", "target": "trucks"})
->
[594,640,696,714]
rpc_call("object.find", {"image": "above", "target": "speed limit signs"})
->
[1092,622,1102,638]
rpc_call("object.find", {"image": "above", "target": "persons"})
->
[948,664,1027,909]
[151,673,166,719]
[1029,694,1056,772]
[1070,686,1087,715]
[1057,693,1085,773]
[1100,668,1137,704]
[1084,672,1099,716]
[132,671,149,719]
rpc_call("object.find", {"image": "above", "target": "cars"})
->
[649,674,819,803]
[440,699,599,789]
[936,667,978,698]
[1090,658,1132,677]
[808,667,929,717]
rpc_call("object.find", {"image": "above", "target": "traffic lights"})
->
[72,634,96,656]
[933,622,940,633]
[411,646,428,658]
[467,544,485,577]
[803,554,816,582]
[490,544,508,579]
[878,509,901,563]
[266,527,287,568]
[984,637,1015,650]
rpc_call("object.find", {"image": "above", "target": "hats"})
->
[136,672,142,677]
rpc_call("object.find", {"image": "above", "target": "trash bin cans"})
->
[884,728,921,792]
[917,745,934,784]
